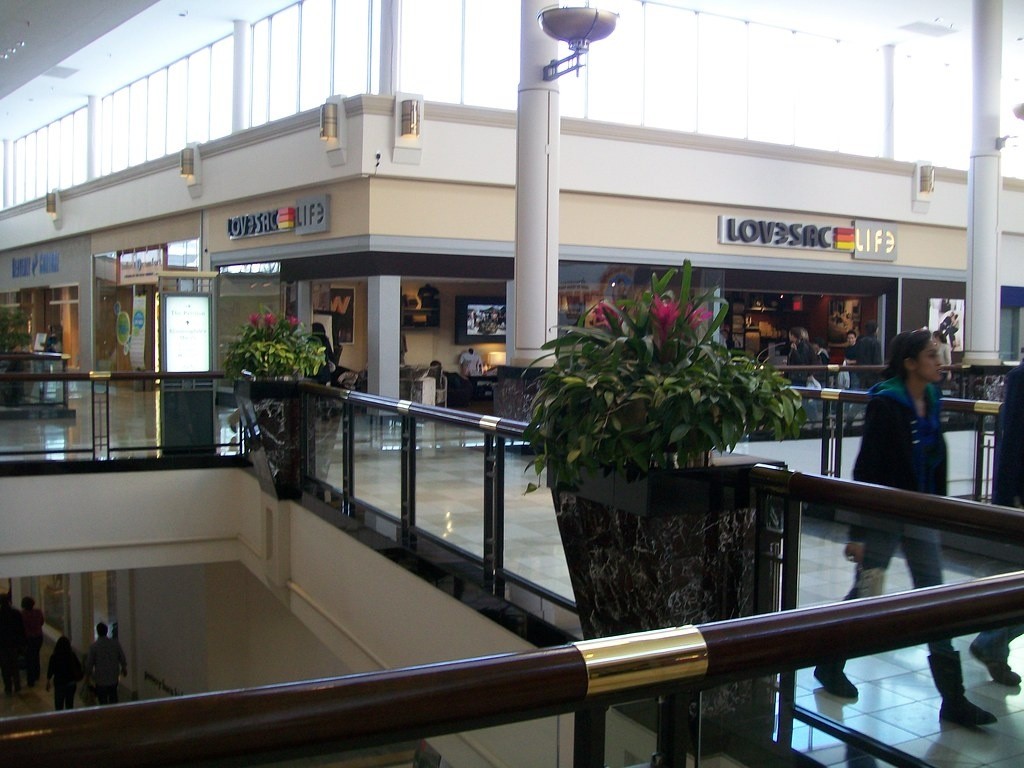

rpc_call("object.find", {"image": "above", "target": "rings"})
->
[848,554,856,565]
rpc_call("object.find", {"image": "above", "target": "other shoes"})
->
[969,643,1021,685]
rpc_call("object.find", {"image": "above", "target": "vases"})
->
[233,368,342,501]
[552,449,793,723]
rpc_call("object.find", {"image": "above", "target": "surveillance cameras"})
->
[375,152,382,159]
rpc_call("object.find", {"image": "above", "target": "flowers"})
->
[517,258,808,494]
[221,311,327,384]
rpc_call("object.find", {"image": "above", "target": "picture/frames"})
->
[456,296,506,344]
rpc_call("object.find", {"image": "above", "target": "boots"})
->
[814,658,859,699]
[927,651,998,725]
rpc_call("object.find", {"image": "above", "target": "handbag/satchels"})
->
[80,682,97,707]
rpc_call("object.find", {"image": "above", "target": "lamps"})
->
[46,192,56,213]
[402,100,421,138]
[996,104,1024,149]
[920,165,934,195]
[535,4,617,81]
[487,351,506,366]
[181,149,194,176]
[319,104,337,140]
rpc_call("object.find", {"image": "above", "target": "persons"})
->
[0,593,128,709]
[971,356,1024,686]
[459,346,484,377]
[813,326,999,725]
[938,298,960,352]
[719,327,732,350]
[304,322,338,386]
[418,283,439,308]
[783,327,884,437]
[744,312,756,329]
[932,330,952,383]
[49,333,63,353]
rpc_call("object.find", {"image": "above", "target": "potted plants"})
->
[0,303,32,405]
